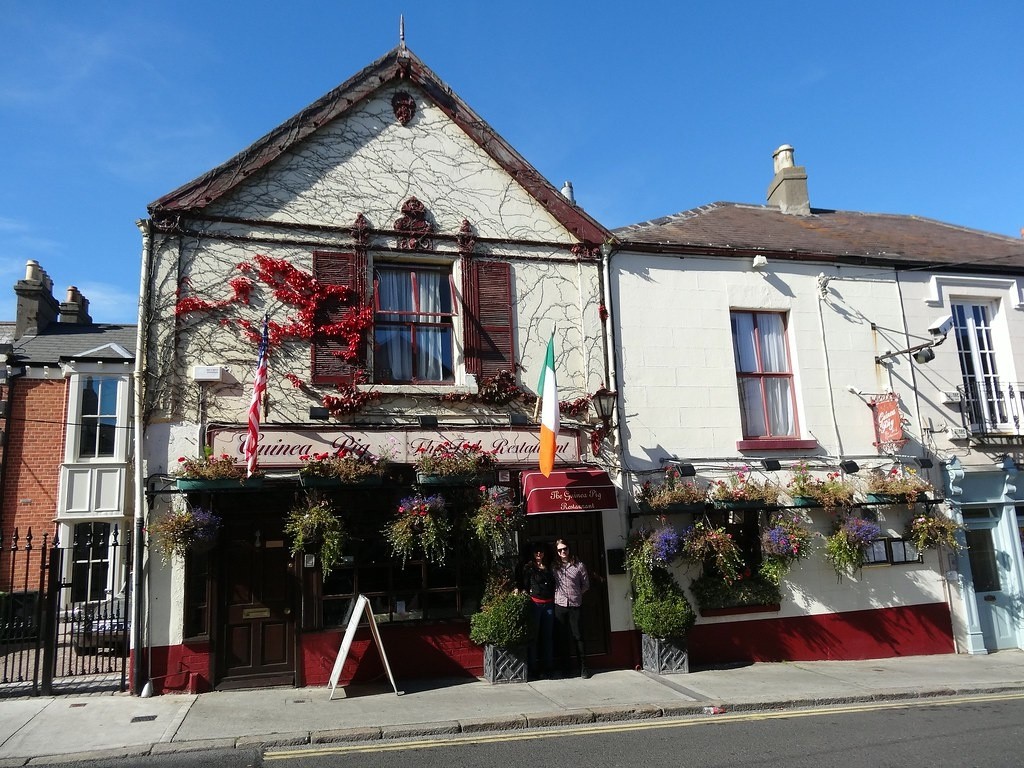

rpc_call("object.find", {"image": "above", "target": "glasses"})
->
[557,547,568,552]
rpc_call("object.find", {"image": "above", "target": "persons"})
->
[520,536,594,682]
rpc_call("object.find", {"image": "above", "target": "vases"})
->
[637,502,705,513]
[711,498,769,511]
[867,493,929,502]
[792,496,821,506]
[418,472,495,486]
[298,471,394,489]
[175,477,264,492]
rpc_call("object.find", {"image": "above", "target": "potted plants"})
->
[621,547,695,674]
[689,566,783,618]
[284,500,347,583]
[467,570,534,683]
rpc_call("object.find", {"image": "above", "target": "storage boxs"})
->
[372,609,424,622]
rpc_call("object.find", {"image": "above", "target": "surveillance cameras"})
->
[927,315,954,335]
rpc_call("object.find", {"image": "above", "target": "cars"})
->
[66,600,109,622]
[70,595,134,658]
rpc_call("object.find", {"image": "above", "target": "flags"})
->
[536,333,560,478]
[243,320,268,478]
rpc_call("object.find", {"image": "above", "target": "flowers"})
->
[176,445,266,486]
[710,466,779,507]
[634,464,707,509]
[638,517,746,585]
[380,490,455,570]
[868,464,938,509]
[786,460,856,514]
[413,438,499,480]
[754,513,817,581]
[464,484,526,561]
[298,439,401,485]
[903,510,972,558]
[140,504,224,574]
[820,515,887,584]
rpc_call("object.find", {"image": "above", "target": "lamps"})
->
[1000,455,1023,473]
[660,458,696,476]
[486,413,527,426]
[820,456,859,474]
[589,381,618,435]
[914,348,935,364]
[892,454,933,470]
[740,457,782,471]
[398,412,439,429]
[309,406,329,421]
[753,255,769,268]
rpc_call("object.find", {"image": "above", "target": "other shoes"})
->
[581,662,592,679]
[529,669,552,679]
[555,666,563,677]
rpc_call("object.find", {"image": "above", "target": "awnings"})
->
[521,467,619,516]
